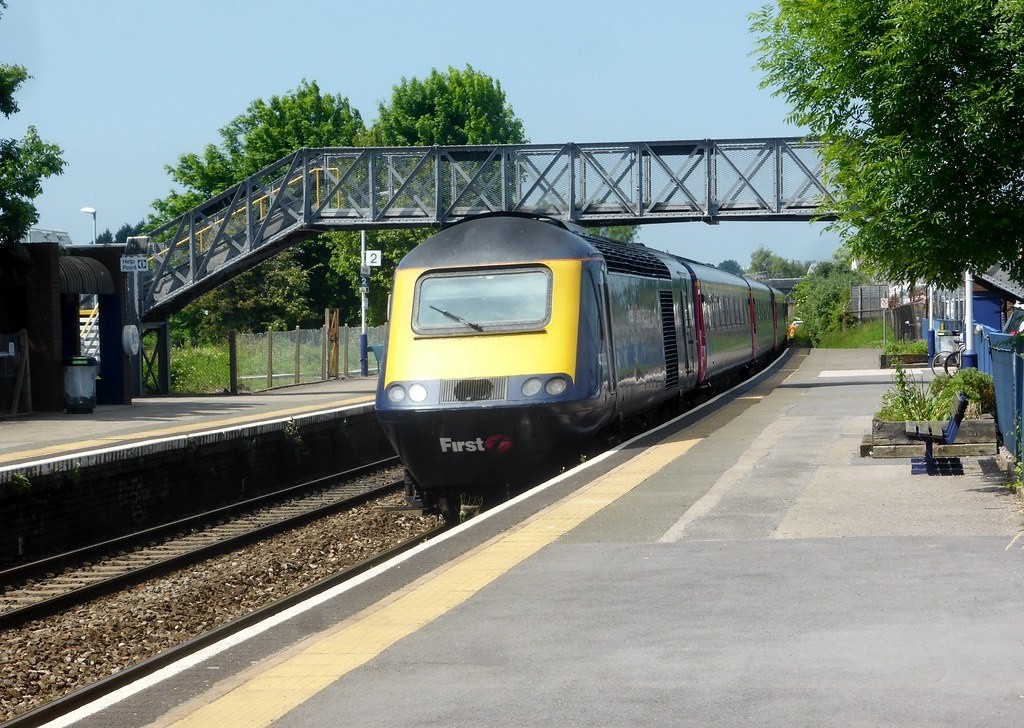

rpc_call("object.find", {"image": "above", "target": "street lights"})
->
[80,206,99,245]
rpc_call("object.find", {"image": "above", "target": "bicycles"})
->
[943,349,962,379]
[930,339,965,379]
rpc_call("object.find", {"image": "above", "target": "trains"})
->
[371,207,789,523]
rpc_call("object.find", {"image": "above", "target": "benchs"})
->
[904,392,969,470]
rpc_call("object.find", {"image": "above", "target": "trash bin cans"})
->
[62,356,98,414]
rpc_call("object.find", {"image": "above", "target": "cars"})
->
[791,321,803,328]
